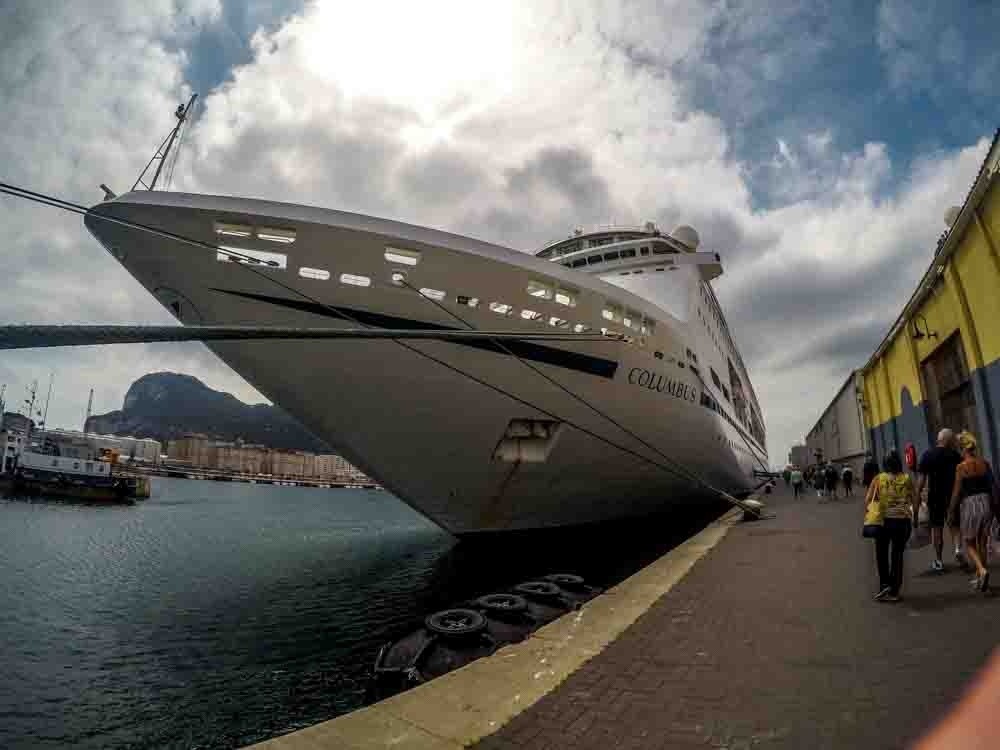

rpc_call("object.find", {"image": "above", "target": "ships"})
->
[83,93,768,553]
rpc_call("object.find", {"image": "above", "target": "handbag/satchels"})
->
[862,478,883,537]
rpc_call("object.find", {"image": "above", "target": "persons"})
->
[912,428,965,571]
[865,450,913,603]
[945,432,996,592]
[782,463,854,503]
[862,454,880,500]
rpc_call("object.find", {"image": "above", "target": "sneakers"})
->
[874,585,899,601]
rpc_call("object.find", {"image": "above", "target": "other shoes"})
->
[953,544,961,560]
[932,559,944,570]
[968,568,990,592]
